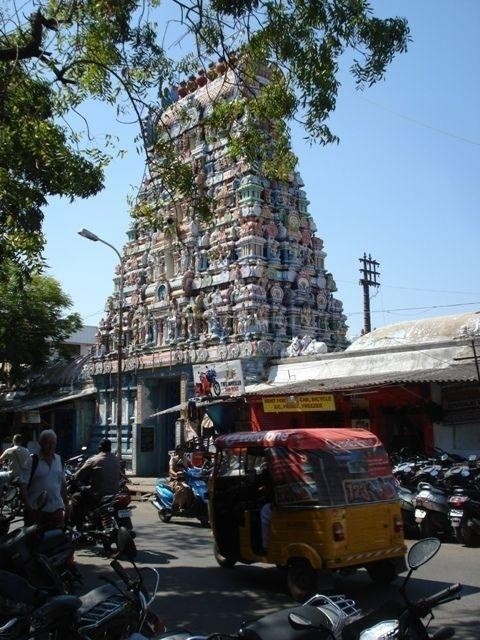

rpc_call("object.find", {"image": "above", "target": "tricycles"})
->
[207,428,407,603]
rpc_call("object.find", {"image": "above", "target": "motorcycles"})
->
[151,457,214,528]
[194,371,214,400]
[0,445,136,559]
[156,590,382,640]
[386,446,480,542]
[0,558,164,639]
[205,365,221,396]
[1,525,86,625]
[287,537,464,640]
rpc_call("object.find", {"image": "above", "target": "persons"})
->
[374,477,395,500]
[252,469,275,555]
[169,445,206,512]
[68,439,120,521]
[0,433,31,487]
[18,429,82,579]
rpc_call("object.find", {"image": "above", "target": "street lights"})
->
[77,227,124,462]
